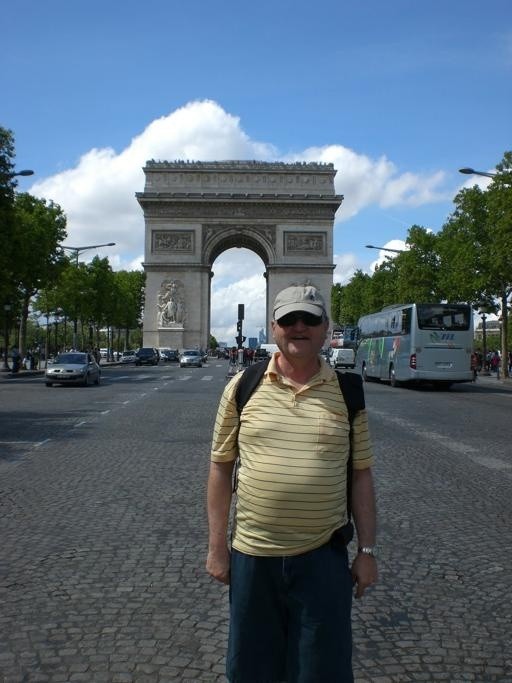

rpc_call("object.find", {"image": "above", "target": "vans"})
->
[330,348,357,369]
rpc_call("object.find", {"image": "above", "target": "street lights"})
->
[459,168,499,180]
[74,243,115,351]
[12,169,34,178]
[365,244,405,256]
[498,318,503,356]
[479,301,488,369]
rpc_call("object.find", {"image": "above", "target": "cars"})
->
[100,348,108,358]
[123,348,207,368]
[44,352,102,387]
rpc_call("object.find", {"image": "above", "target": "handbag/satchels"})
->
[330,524,354,553]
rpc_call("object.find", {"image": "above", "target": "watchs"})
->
[356,542,379,558]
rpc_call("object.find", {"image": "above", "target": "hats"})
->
[272,286,326,321]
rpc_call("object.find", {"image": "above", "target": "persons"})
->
[157,279,187,327]
[22,341,41,371]
[205,284,380,683]
[11,342,23,373]
[472,345,511,378]
[47,345,102,366]
[205,346,257,367]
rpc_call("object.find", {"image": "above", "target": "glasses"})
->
[277,313,322,327]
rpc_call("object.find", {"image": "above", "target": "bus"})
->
[350,302,475,390]
